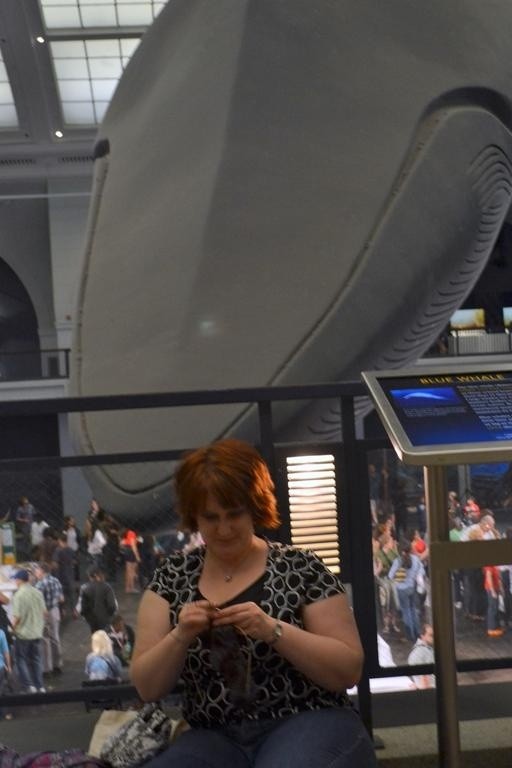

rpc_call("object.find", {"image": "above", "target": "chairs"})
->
[82,678,126,714]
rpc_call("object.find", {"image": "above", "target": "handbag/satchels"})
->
[100,701,173,768]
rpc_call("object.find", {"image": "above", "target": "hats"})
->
[10,571,29,582]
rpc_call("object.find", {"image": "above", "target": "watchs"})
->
[265,617,284,646]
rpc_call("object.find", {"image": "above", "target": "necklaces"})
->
[200,537,259,582]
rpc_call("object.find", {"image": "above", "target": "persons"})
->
[368,466,508,690]
[0,496,204,695]
[129,438,379,765]
